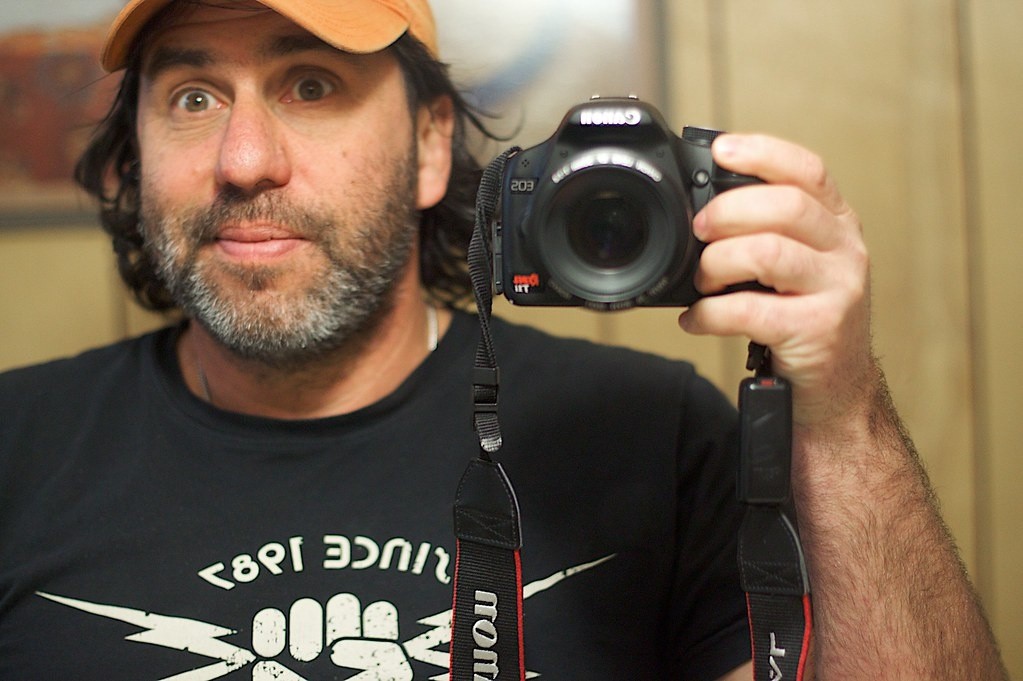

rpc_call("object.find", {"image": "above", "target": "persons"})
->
[0,0,1010,681]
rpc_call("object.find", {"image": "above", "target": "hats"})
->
[103,0,437,69]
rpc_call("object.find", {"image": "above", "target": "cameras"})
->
[493,94,779,314]
